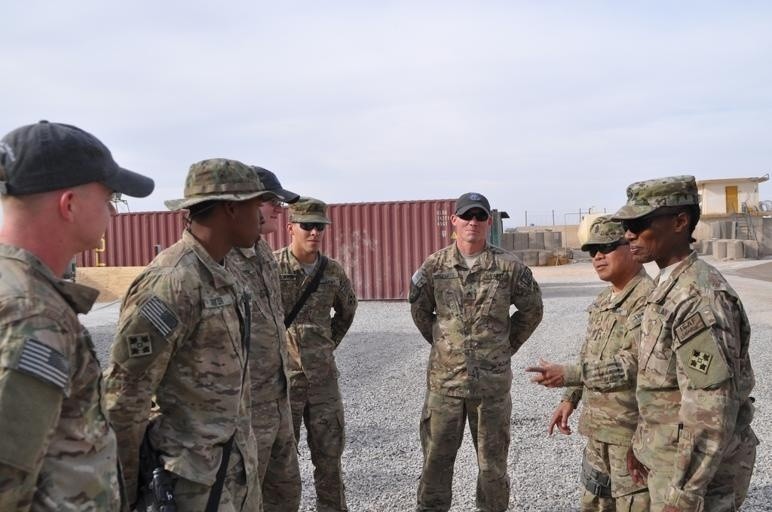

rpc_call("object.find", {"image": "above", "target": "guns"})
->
[134,430,177,511]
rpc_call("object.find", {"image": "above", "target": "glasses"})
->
[300,222,324,231]
[459,211,488,221]
[589,242,629,257]
[622,213,677,234]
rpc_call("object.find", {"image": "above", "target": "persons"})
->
[0,121,154,512]
[224,167,302,512]
[273,197,359,512]
[525,216,656,512]
[612,176,760,512]
[100,158,285,512]
[409,194,544,512]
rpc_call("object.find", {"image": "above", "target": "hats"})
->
[582,176,697,252]
[0,121,154,197]
[455,193,490,216]
[164,159,333,225]
